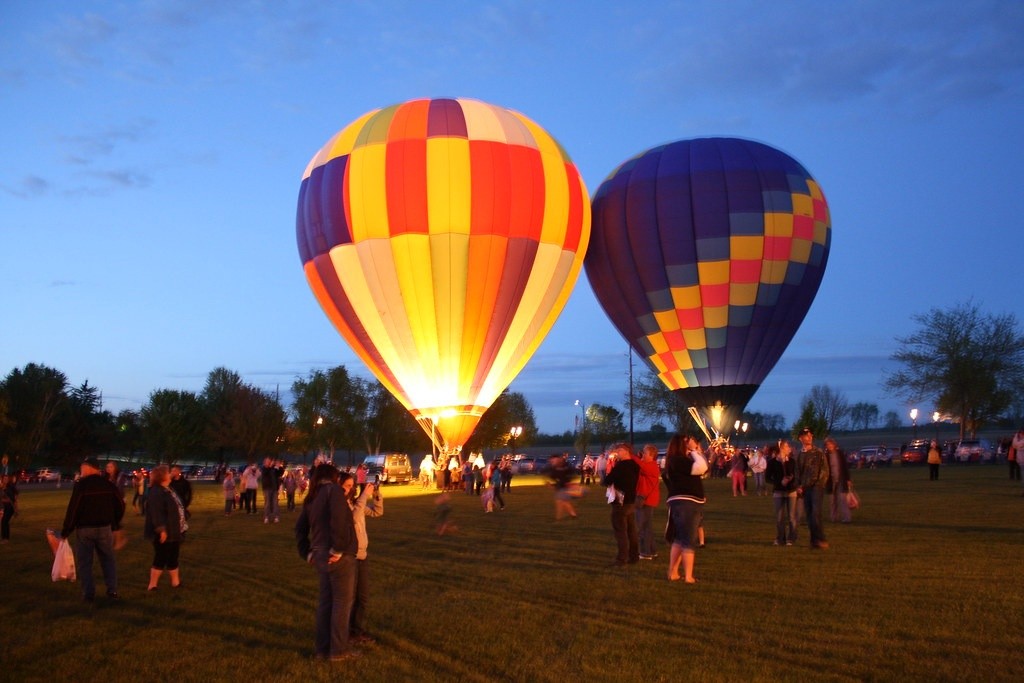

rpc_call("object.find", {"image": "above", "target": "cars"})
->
[494,454,600,473]
[180,464,238,476]
[128,466,151,479]
[28,470,61,482]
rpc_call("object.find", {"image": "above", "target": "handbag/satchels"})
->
[846,487,860,509]
[51,537,76,582]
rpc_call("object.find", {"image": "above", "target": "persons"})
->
[797,426,830,548]
[0,473,19,545]
[702,443,770,497]
[419,451,514,514]
[766,439,801,546]
[295,464,364,661]
[223,456,370,523]
[132,465,193,542]
[1007,429,1024,481]
[579,443,660,568]
[337,471,383,646]
[848,451,876,471]
[431,492,459,537]
[926,439,943,481]
[142,465,189,591]
[824,437,853,523]
[60,457,127,613]
[544,452,577,521]
[661,431,715,585]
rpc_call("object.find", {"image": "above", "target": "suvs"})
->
[899,438,997,466]
[848,449,894,469]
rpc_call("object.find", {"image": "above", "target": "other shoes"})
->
[264,518,268,523]
[811,539,829,549]
[314,651,361,661]
[108,592,118,601]
[613,554,657,566]
[82,594,95,603]
[773,541,779,545]
[786,542,792,545]
[347,633,375,644]
[274,517,279,522]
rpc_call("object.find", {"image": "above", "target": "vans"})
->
[362,453,412,486]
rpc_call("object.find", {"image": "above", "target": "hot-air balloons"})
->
[295,95,593,490]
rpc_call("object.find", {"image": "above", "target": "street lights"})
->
[582,136,832,480]
[734,420,749,447]
[910,408,917,439]
[511,426,522,457]
[932,411,942,440]
[574,399,585,455]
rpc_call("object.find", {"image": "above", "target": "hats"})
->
[82,455,99,469]
